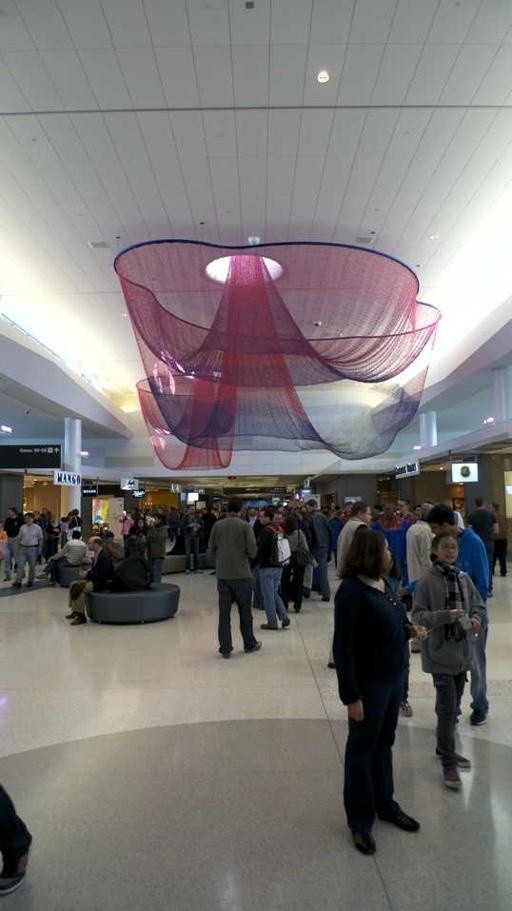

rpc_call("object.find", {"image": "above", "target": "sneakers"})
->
[219,642,261,659]
[436,747,470,787]
[261,619,290,629]
[253,585,329,612]
[0,845,28,895]
[66,612,86,625]
[471,708,487,725]
[4,556,55,587]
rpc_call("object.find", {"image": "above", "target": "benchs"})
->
[194,553,210,568]
[59,565,84,587]
[85,583,180,626]
[162,554,186,575]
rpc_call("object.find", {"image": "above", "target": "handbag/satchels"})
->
[293,542,307,566]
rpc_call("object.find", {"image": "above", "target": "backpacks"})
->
[264,525,292,566]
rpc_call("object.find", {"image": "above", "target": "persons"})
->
[208,499,262,657]
[1,507,87,587]
[1,785,31,893]
[332,529,426,854]
[415,535,487,790]
[65,498,330,626]
[329,498,509,725]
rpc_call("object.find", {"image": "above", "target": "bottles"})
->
[459,614,478,642]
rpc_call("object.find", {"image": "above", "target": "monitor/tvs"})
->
[449,462,481,485]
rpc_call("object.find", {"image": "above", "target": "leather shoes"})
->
[353,828,375,853]
[377,806,419,831]
